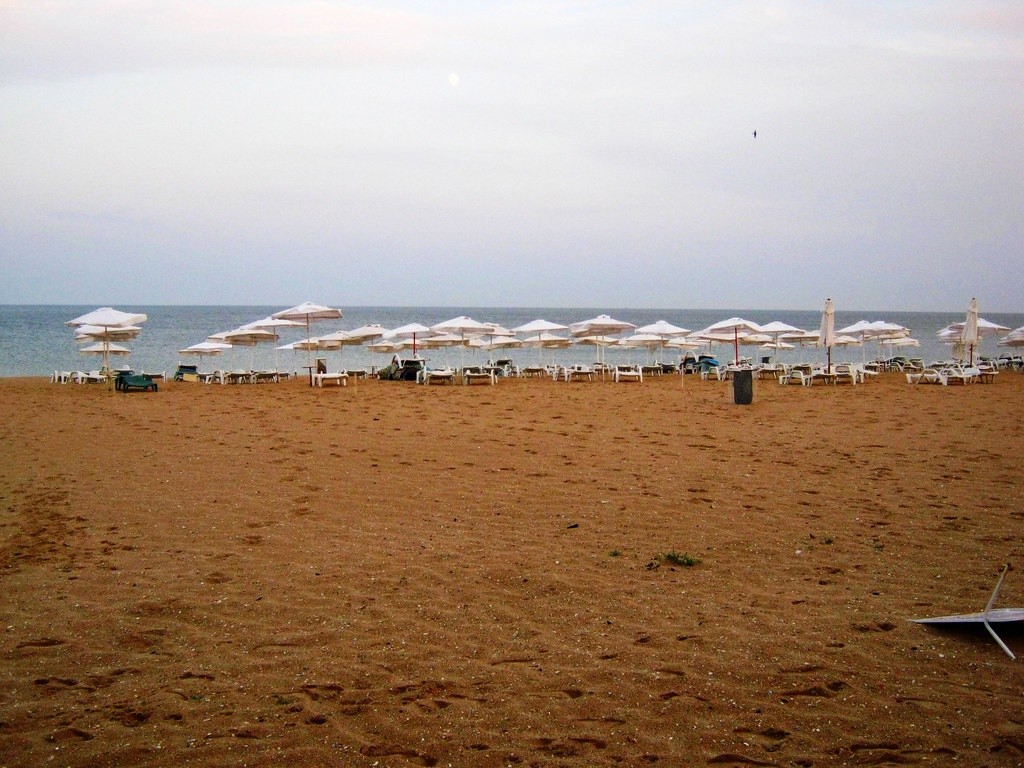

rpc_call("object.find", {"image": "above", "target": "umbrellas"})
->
[64,306,148,390]
[996,326,1024,347]
[819,298,835,385]
[277,316,573,384]
[567,315,637,382]
[272,302,343,370]
[935,317,1011,365]
[809,319,920,369]
[961,297,977,383]
[179,316,307,382]
[626,318,821,375]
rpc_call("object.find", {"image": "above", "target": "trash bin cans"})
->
[761,356,770,363]
[732,369,753,403]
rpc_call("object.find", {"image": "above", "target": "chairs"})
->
[313,373,348,387]
[51,368,166,392]
[175,363,290,385]
[680,351,1024,386]
[459,358,677,385]
[341,369,367,378]
[391,352,458,385]
